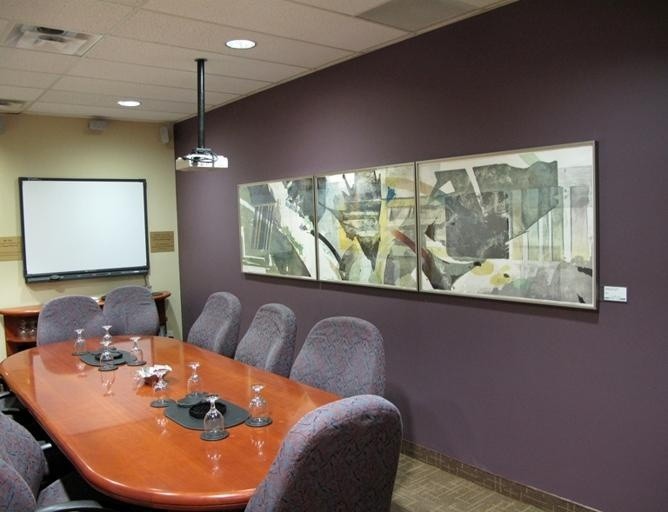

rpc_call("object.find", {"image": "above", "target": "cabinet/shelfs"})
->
[0,290,173,358]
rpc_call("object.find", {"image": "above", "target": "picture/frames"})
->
[236,173,318,283]
[416,141,597,310]
[318,162,419,291]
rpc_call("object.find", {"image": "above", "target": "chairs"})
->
[290,317,391,398]
[187,292,242,359]
[38,295,106,341]
[235,302,298,379]
[103,286,161,334]
[243,394,403,512]
[0,378,103,512]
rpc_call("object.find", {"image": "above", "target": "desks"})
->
[0,334,343,512]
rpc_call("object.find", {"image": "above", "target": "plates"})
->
[150,399,169,407]
[246,417,272,427]
[98,367,118,370]
[72,351,90,356]
[200,431,229,441]
[127,361,146,365]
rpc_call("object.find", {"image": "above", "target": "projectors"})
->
[175,153,229,172]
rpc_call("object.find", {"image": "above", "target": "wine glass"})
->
[185,362,205,401]
[127,336,144,365]
[203,396,225,438]
[247,383,271,424]
[151,368,169,404]
[99,340,115,369]
[102,326,113,342]
[73,329,87,354]
